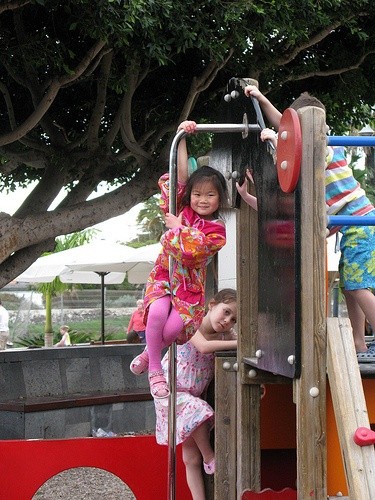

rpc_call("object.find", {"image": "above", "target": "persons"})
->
[60,325,70,345]
[245,85,375,353]
[128,120,227,398]
[0,299,9,350]
[235,169,257,210]
[153,289,237,500]
[127,300,146,343]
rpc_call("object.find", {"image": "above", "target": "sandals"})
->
[148,369,170,399]
[130,345,149,375]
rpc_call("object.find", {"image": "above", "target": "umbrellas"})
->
[12,238,156,345]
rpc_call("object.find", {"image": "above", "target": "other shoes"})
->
[204,458,214,475]
